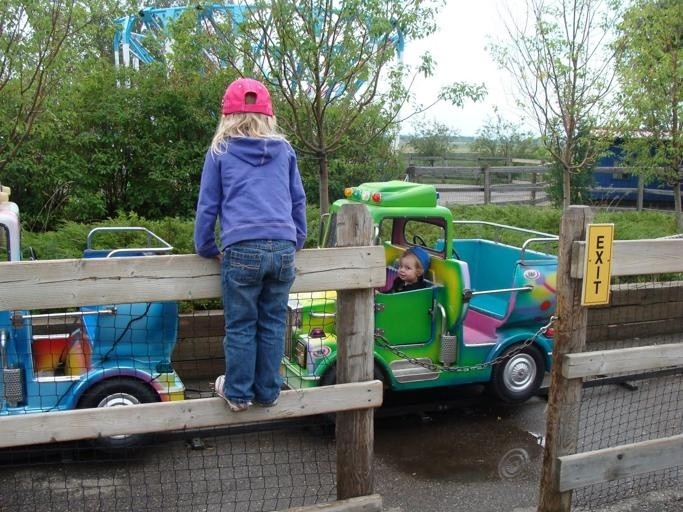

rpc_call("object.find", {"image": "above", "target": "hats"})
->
[223,78,274,115]
[408,247,430,272]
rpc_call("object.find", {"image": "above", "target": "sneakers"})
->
[214,375,248,411]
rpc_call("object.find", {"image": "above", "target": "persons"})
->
[384,247,431,293]
[195,78,307,413]
